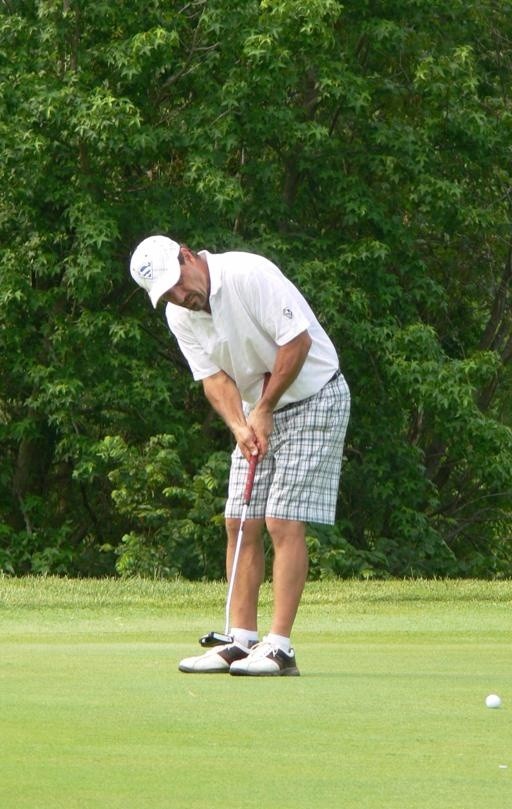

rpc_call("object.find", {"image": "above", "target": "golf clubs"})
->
[199,371,272,648]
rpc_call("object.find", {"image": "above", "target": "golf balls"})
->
[485,694,501,709]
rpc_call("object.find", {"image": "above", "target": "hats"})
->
[127,234,183,311]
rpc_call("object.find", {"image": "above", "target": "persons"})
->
[127,230,354,678]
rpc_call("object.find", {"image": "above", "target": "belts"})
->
[328,368,341,384]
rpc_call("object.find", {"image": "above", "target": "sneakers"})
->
[177,640,251,674]
[229,637,299,679]
